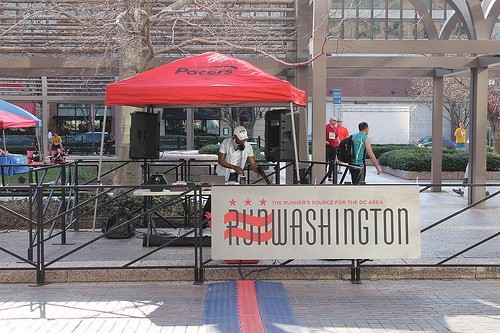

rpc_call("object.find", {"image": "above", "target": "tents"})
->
[90,51,307,250]
[0,99,43,182]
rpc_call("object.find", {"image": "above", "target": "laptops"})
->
[200,175,225,185]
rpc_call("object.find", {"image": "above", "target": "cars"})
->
[62,132,115,155]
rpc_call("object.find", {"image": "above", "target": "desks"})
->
[133,187,211,245]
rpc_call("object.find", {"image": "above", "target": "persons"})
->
[0,128,116,167]
[198,126,264,235]
[335,117,349,174]
[454,122,467,151]
[348,121,382,184]
[418,140,422,146]
[326,116,340,181]
[452,162,490,197]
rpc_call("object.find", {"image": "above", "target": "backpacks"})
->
[337,135,354,163]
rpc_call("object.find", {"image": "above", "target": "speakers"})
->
[265,109,299,163]
[130,111,160,159]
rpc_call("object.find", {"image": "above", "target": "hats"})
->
[234,126,248,140]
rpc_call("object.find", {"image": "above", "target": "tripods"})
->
[128,159,178,235]
